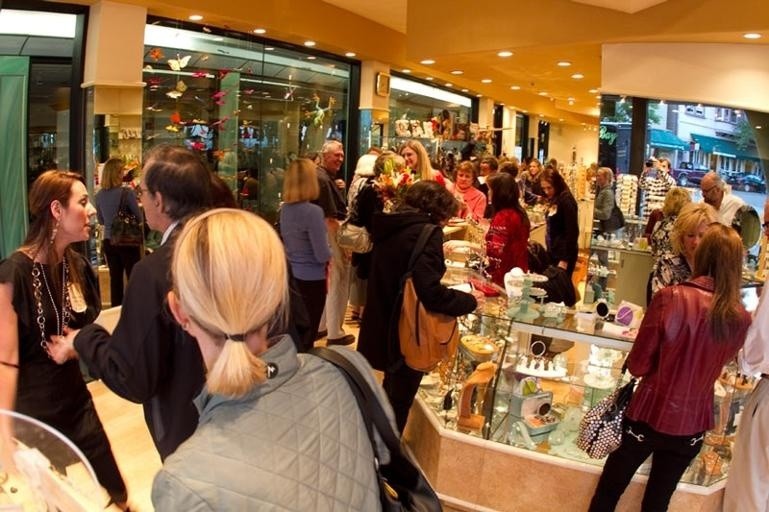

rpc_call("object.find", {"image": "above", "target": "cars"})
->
[727,172,766,195]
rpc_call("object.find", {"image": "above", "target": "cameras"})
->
[645,159,653,168]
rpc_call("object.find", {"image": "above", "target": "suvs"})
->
[670,161,710,187]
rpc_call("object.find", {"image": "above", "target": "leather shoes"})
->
[314,330,355,345]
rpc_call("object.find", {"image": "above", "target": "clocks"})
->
[376,72,390,98]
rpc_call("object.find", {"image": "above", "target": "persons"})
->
[148,207,401,512]
[1,139,482,462]
[585,221,768,512]
[635,154,744,317]
[471,156,617,354]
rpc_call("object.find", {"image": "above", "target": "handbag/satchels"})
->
[600,207,623,231]
[398,222,460,373]
[336,221,373,254]
[577,378,636,458]
[309,346,443,512]
[111,187,142,246]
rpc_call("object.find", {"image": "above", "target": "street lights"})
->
[694,143,700,163]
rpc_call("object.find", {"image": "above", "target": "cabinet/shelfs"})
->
[411,208,761,511]
